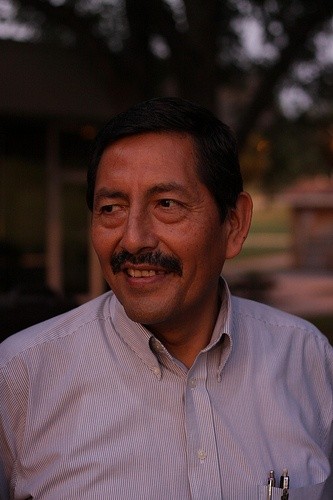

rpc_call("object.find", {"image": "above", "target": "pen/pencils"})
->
[280,468,290,489]
[281,489,289,500]
[267,470,275,500]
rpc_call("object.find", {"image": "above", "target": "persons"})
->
[0,94,332,500]
